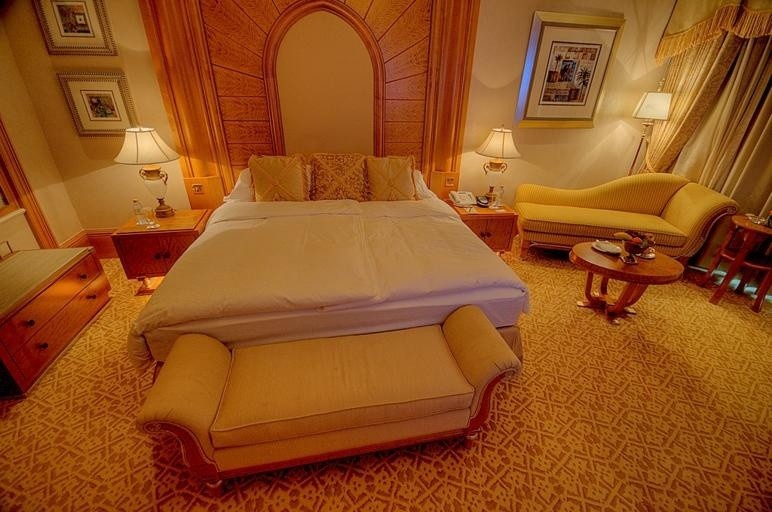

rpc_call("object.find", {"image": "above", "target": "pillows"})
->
[223,152,434,204]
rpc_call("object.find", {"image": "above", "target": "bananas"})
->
[614,232,633,240]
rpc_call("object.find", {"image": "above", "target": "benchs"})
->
[132,303,524,500]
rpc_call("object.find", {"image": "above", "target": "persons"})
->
[560,64,571,81]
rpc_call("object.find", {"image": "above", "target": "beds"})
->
[129,163,531,387]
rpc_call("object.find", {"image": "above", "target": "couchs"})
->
[510,172,740,263]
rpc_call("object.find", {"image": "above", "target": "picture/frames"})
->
[512,10,628,131]
[55,70,141,138]
[32,1,123,58]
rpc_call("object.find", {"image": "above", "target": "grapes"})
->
[625,229,645,239]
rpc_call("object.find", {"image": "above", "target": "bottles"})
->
[132,199,147,226]
[495,185,504,207]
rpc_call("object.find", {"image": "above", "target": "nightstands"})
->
[443,197,518,258]
[110,208,213,296]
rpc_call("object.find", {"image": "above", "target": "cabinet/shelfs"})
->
[1,245,111,402]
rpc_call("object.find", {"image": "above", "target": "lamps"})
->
[627,77,674,175]
[113,126,183,219]
[474,123,523,207]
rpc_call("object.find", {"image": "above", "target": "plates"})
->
[591,241,622,254]
[636,251,656,259]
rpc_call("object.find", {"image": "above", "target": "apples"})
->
[645,233,654,241]
[632,237,643,246]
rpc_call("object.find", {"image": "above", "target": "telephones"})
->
[448,190,477,206]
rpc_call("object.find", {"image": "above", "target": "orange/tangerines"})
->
[643,241,648,246]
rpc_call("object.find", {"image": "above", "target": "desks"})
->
[694,213,771,313]
[568,240,686,326]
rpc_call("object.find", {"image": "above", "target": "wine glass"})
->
[141,207,160,228]
[619,240,647,264]
